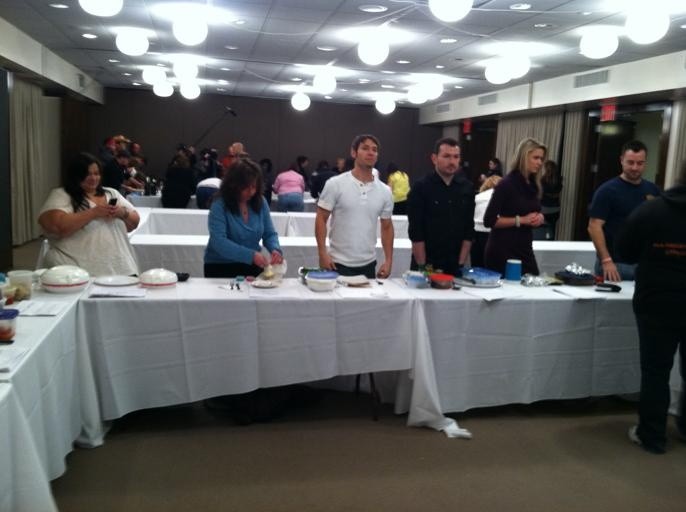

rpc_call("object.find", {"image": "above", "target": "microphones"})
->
[225,105,237,117]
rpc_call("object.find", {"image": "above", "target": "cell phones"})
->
[108,198,117,206]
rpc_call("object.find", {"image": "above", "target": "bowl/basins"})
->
[303,273,336,292]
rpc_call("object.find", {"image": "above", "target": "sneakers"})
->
[627,424,643,445]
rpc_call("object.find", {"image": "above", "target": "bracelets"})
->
[599,256,613,266]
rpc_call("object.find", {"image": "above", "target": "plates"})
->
[40,265,89,293]
[139,268,177,288]
[251,281,276,288]
[93,275,139,286]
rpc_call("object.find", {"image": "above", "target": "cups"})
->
[505,259,521,283]
[7,270,32,298]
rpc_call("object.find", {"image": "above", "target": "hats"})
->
[114,136,130,143]
[229,143,248,156]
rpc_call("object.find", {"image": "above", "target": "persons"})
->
[586,140,662,286]
[33,149,140,277]
[99,132,565,241]
[204,157,283,278]
[312,134,396,280]
[621,162,686,456]
[481,136,549,276]
[407,136,477,269]
